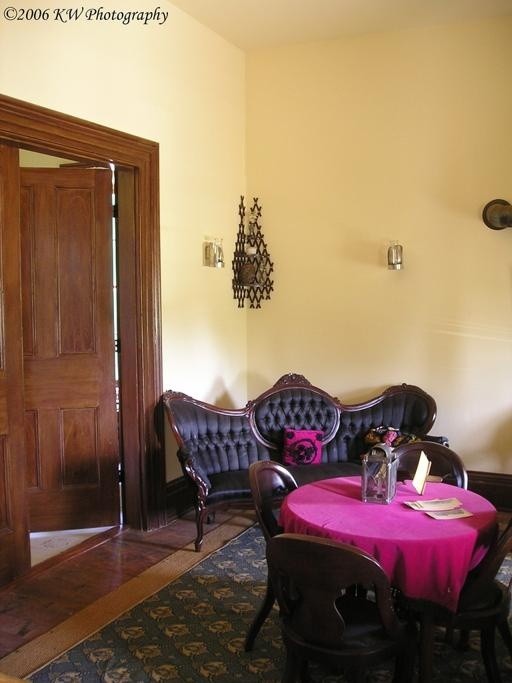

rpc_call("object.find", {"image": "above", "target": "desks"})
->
[278,474,500,653]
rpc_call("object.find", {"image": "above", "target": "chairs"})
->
[381,442,468,647]
[388,522,512,681]
[265,531,419,683]
[245,460,298,652]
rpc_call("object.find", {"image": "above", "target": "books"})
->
[411,449,432,494]
[425,507,475,520]
[417,496,464,507]
[426,474,446,482]
[404,497,443,510]
[414,501,461,509]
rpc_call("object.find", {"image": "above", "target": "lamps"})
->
[203,236,225,269]
[386,239,404,271]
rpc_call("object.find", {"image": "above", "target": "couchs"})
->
[159,373,449,553]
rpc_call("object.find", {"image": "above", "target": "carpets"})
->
[0,503,512,683]
